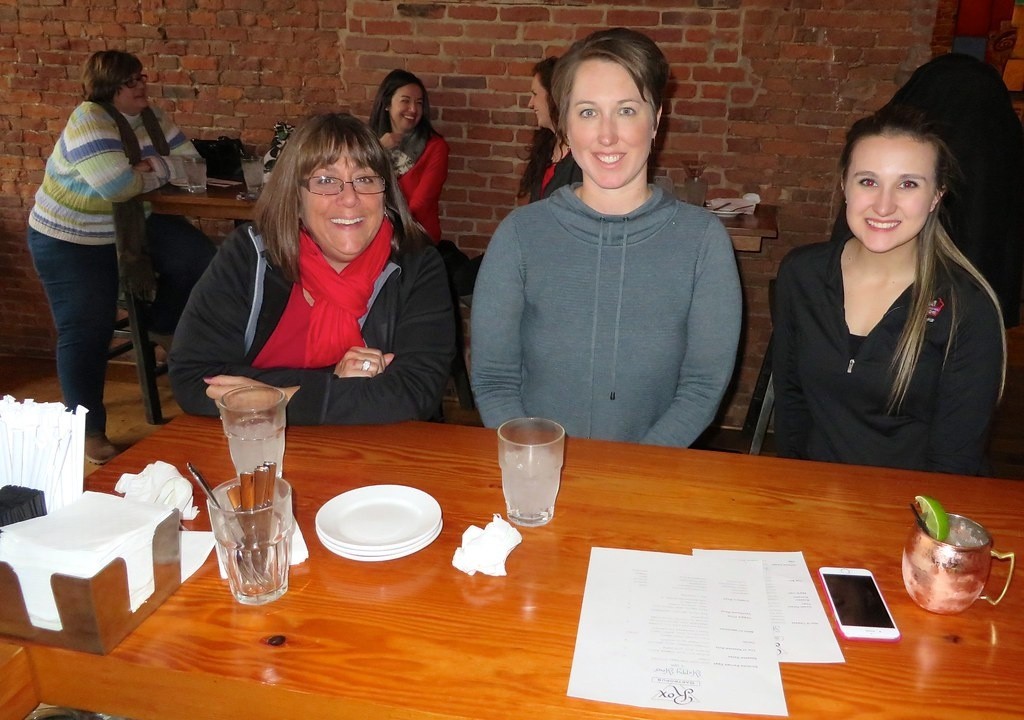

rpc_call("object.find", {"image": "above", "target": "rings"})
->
[362,360,371,371]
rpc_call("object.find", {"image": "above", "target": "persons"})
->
[468,26,744,449]
[516,56,583,204]
[771,103,1007,479]
[829,53,1024,329]
[367,68,470,423]
[26,49,202,464]
[167,112,457,425]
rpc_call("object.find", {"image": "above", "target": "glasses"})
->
[298,176,386,195]
[120,73,148,88]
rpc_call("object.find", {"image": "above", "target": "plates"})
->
[314,484,442,561]
[709,209,742,217]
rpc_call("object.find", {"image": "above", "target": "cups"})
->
[681,160,708,179]
[497,417,565,526]
[685,177,707,206]
[241,157,264,193]
[902,513,1014,615]
[207,476,293,604]
[183,157,206,193]
[219,386,286,479]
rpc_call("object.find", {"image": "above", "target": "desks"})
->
[719,203,778,251]
[0,414,1024,720]
[136,178,261,230]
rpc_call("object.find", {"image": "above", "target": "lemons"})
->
[914,495,949,542]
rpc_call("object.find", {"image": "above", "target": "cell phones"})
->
[818,566,901,642]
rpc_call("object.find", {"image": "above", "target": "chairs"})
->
[105,197,169,424]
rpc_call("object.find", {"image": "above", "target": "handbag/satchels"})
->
[191,136,250,182]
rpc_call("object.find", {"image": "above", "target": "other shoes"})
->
[85,433,121,464]
[154,344,168,365]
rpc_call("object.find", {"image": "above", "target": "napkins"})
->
[452,513,522,577]
[205,498,309,579]
[1,491,217,631]
[115,461,200,520]
[710,190,761,215]
